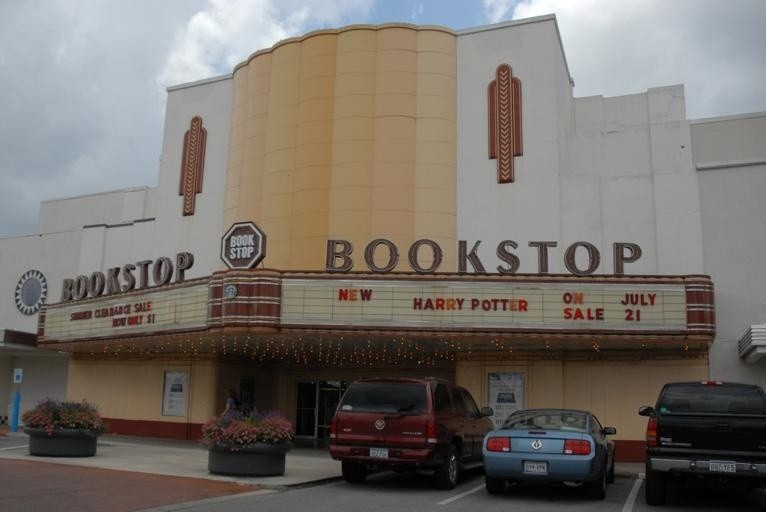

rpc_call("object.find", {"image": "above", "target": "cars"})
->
[638,381,765,506]
[327,375,494,489]
[481,408,616,501]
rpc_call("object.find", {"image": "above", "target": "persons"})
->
[219,388,240,418]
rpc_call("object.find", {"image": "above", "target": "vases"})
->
[208,443,290,476]
[23,425,98,458]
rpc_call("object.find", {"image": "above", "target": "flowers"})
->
[21,395,105,438]
[200,406,297,453]
